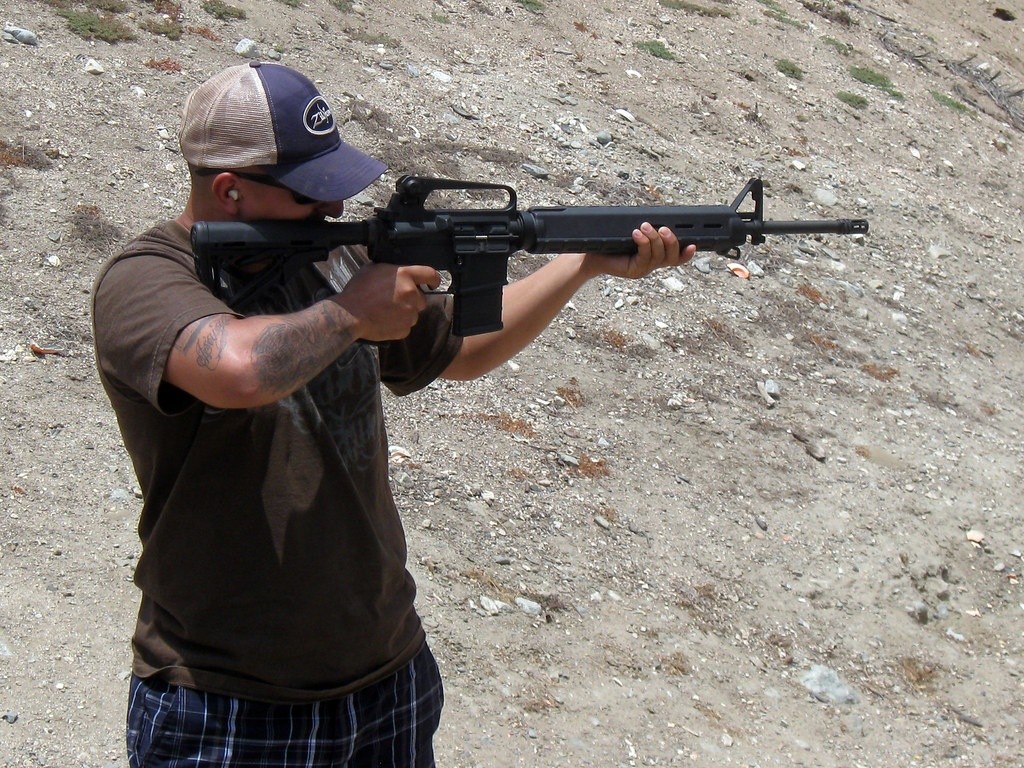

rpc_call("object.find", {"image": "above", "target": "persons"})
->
[90,61,694,768]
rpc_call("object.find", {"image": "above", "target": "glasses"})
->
[195,167,321,205]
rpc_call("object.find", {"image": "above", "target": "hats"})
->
[178,60,388,204]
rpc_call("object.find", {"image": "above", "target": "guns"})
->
[188,172,870,339]
